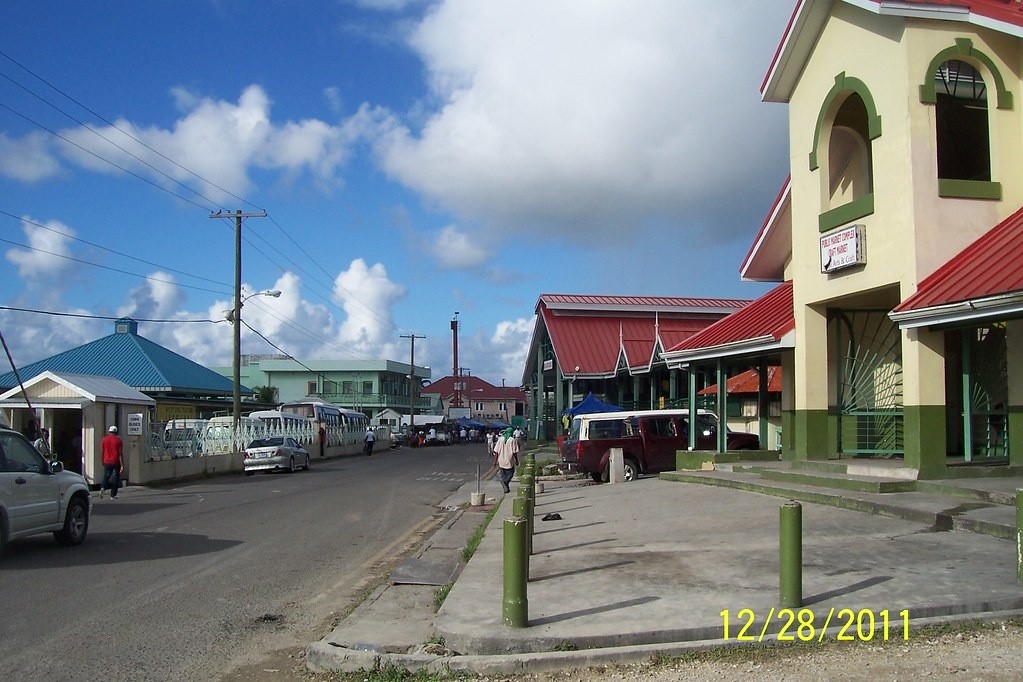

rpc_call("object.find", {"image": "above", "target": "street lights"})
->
[233,289,281,450]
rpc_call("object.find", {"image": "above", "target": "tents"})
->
[560,391,625,414]
[455,416,511,430]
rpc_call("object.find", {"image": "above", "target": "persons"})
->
[484,430,499,456]
[418,429,425,446]
[429,426,436,445]
[23,420,38,441]
[365,428,375,456]
[100,425,124,500]
[460,427,481,445]
[319,427,326,457]
[35,430,50,457]
[514,426,523,451]
[493,427,519,493]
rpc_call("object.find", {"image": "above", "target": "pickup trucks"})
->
[560,414,758,481]
[426,429,453,446]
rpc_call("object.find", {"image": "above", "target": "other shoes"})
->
[110,496,119,500]
[368,453,372,456]
[501,481,509,491]
[100,488,105,498]
[504,490,510,493]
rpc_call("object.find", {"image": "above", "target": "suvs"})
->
[0,423,89,550]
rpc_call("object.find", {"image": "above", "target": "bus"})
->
[277,398,340,427]
[340,409,368,430]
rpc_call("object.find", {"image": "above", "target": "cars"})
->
[377,426,403,444]
[242,436,310,476]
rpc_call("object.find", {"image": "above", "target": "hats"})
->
[109,426,118,432]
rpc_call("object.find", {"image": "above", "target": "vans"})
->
[163,420,205,458]
[571,410,732,439]
[249,410,309,444]
[203,416,260,454]
